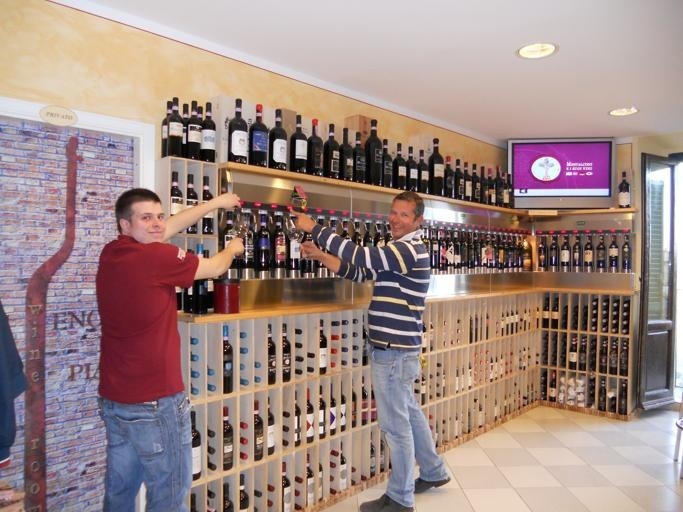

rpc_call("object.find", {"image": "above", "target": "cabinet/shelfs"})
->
[536,207,637,422]
[155,155,536,512]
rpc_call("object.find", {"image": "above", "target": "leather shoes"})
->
[414,476,451,493]
[359,493,414,512]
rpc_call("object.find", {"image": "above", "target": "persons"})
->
[95,188,245,512]
[290,190,452,512]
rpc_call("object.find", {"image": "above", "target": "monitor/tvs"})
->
[507,138,617,209]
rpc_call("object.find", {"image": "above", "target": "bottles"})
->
[162,97,632,512]
